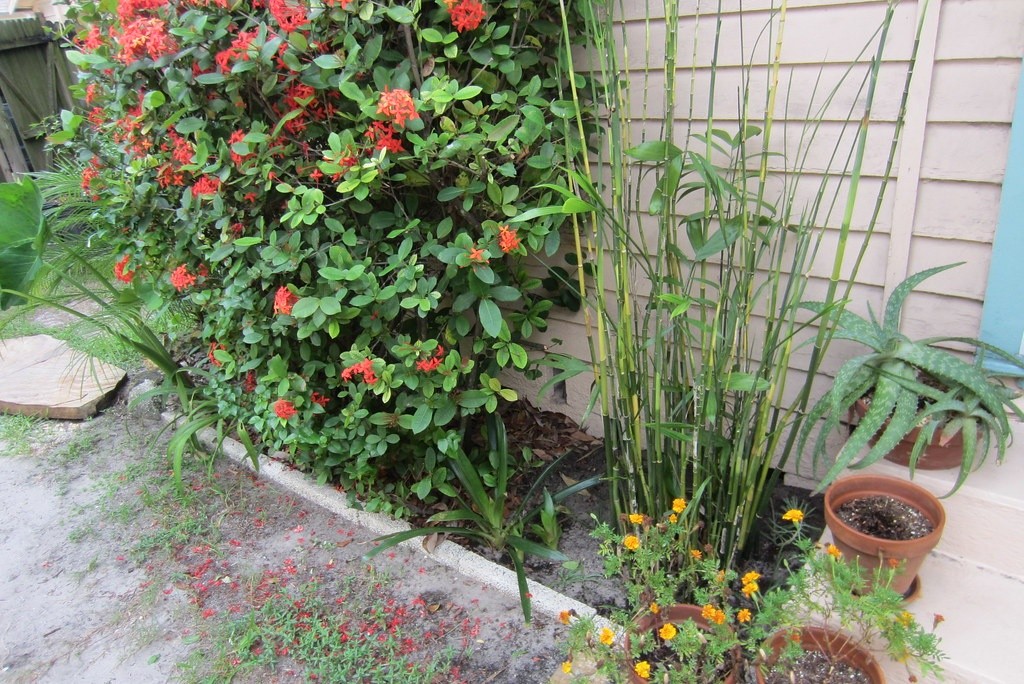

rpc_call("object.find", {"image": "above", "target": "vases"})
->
[822,472,946,611]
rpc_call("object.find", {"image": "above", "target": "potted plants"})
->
[556,498,744,684]
[778,261,1024,499]
[737,495,951,684]
